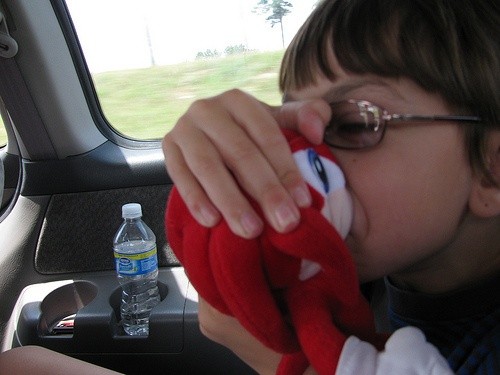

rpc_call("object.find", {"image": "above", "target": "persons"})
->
[4,0,500,375]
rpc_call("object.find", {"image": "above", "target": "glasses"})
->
[322,99,482,151]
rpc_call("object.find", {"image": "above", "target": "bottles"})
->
[113,203,161,335]
